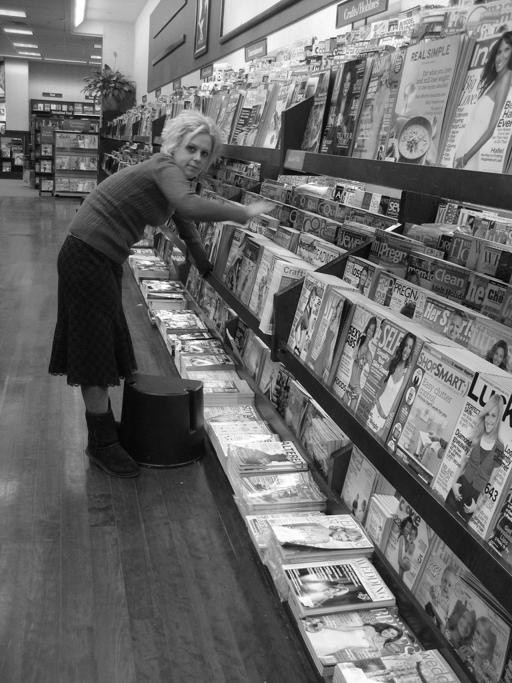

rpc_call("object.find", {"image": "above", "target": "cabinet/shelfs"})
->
[1,85,99,197]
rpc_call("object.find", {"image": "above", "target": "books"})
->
[0,81,129,199]
[130,3,511,682]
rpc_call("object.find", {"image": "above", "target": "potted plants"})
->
[79,60,137,116]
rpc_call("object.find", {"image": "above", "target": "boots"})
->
[83,394,144,480]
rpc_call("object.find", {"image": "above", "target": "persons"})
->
[46,105,280,478]
